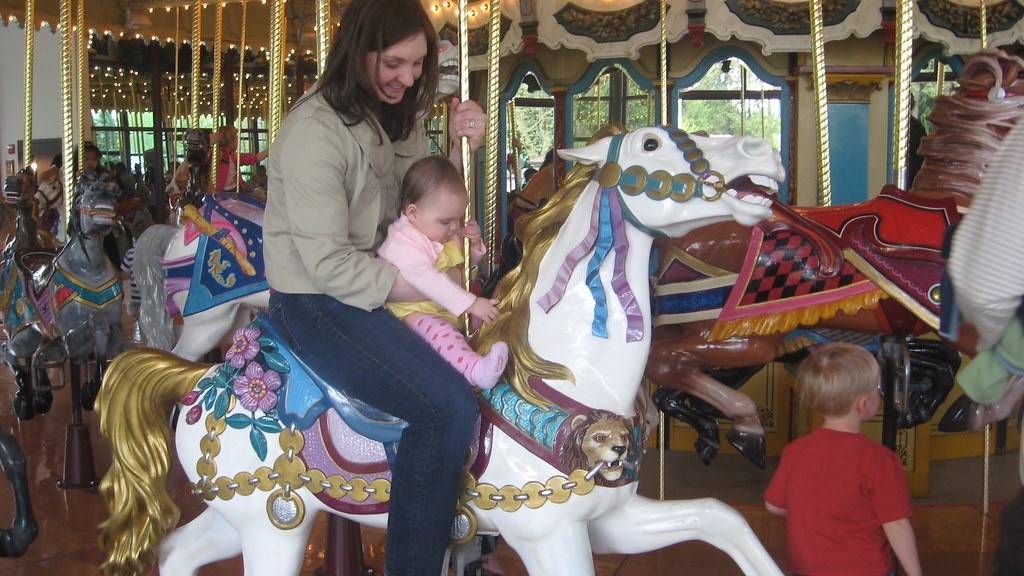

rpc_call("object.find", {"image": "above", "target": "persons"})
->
[905,92,1023,430]
[521,169,538,190]
[376,155,509,390]
[33,126,269,318]
[763,342,923,576]
[262,1,486,576]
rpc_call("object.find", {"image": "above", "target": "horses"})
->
[0,47,1023,576]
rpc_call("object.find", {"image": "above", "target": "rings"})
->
[469,120,475,128]
